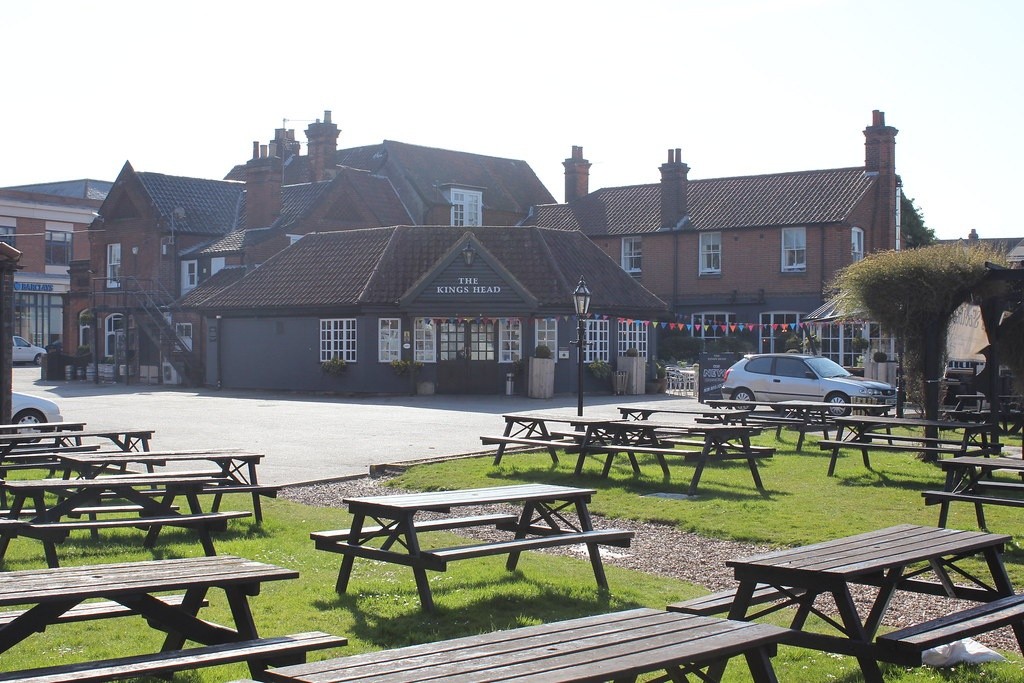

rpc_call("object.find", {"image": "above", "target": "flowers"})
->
[390,359,424,377]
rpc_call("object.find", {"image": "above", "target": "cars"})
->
[12,336,48,365]
[720,354,896,416]
[11,391,63,442]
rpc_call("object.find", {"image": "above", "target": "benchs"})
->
[0,393,1024,683]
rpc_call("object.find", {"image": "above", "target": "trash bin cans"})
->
[41,354,65,381]
[611,370,629,394]
[506,373,514,395]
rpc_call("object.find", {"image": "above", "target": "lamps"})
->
[462,240,475,266]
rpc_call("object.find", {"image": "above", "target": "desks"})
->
[266,606,793,683]
[0,475,230,568]
[335,483,609,621]
[51,449,266,526]
[0,554,300,683]
[703,523,1024,683]
[827,414,994,479]
[937,456,1024,533]
[600,420,767,496]
[0,421,87,446]
[0,430,158,491]
[704,400,892,452]
[493,411,629,481]
[600,406,768,481]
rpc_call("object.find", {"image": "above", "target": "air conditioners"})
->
[162,361,186,384]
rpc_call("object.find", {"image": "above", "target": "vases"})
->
[401,369,420,377]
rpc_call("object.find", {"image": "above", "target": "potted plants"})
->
[874,352,898,389]
[646,379,660,394]
[104,356,115,384]
[73,344,93,364]
[617,347,645,395]
[98,359,104,376]
[652,360,667,393]
[528,342,555,399]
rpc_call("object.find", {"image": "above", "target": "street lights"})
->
[570,275,592,443]
